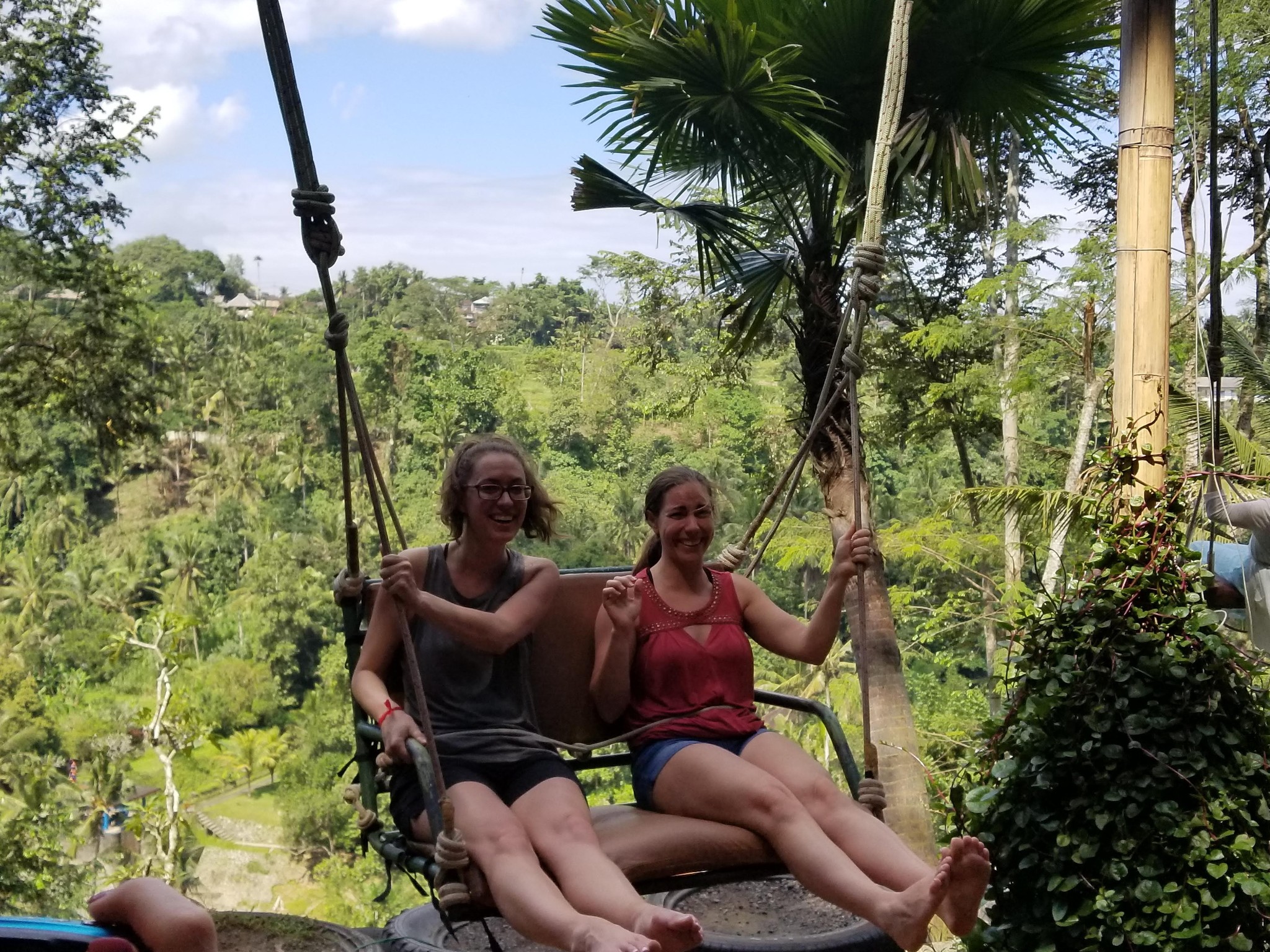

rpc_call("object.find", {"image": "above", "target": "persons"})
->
[1189,441,1270,596]
[590,466,990,952]
[352,433,705,952]
[88,877,218,952]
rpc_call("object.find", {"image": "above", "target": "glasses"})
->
[467,483,533,502]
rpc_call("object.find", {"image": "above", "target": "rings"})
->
[869,547,874,554]
[388,576,393,586]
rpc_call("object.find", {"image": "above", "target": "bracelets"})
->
[378,698,403,728]
